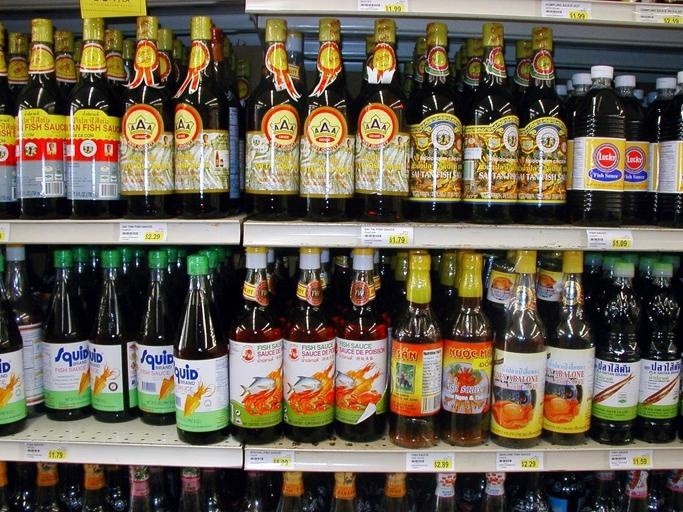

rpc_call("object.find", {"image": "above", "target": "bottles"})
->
[0,453,682,511]
[0,247,683,453]
[0,13,683,231]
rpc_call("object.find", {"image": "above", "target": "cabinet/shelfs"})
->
[0,3,682,470]
